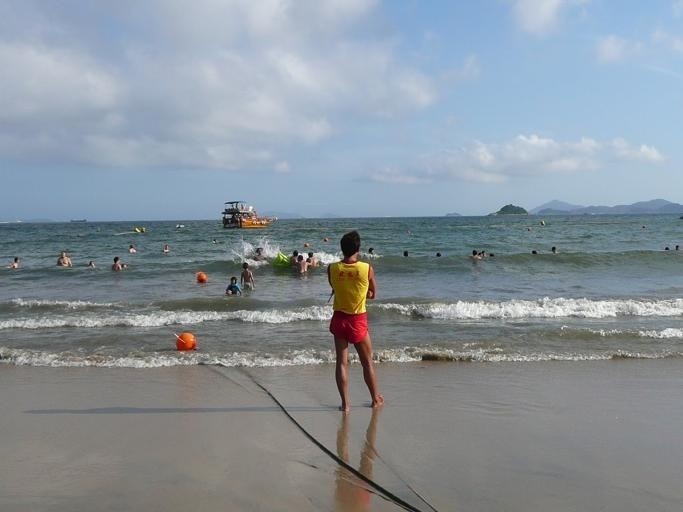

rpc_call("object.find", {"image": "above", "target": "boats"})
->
[221,199,279,229]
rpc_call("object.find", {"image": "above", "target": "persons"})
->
[112,257,121,271]
[58,251,72,267]
[676,245,679,250]
[532,250,537,254]
[368,248,376,254]
[291,250,318,273]
[88,261,95,268]
[327,229,384,411]
[241,262,256,289]
[129,245,136,254]
[665,247,670,250]
[552,247,556,253]
[404,251,408,256]
[225,277,242,297]
[436,253,441,256]
[471,249,494,259]
[254,248,265,261]
[163,245,170,253]
[12,257,19,268]
